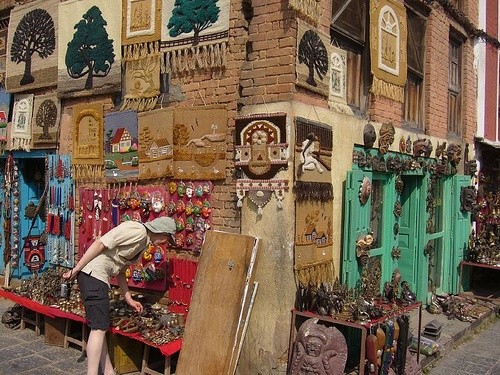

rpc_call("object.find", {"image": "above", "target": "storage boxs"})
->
[44,314,69,347]
[107,332,157,374]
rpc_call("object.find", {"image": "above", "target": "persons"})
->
[300,133,324,175]
[290,335,337,375]
[63,216,176,375]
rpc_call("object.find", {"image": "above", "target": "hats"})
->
[143,217,177,246]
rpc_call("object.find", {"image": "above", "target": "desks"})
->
[460,263,500,301]
[287,301,423,375]
[0,289,184,375]
